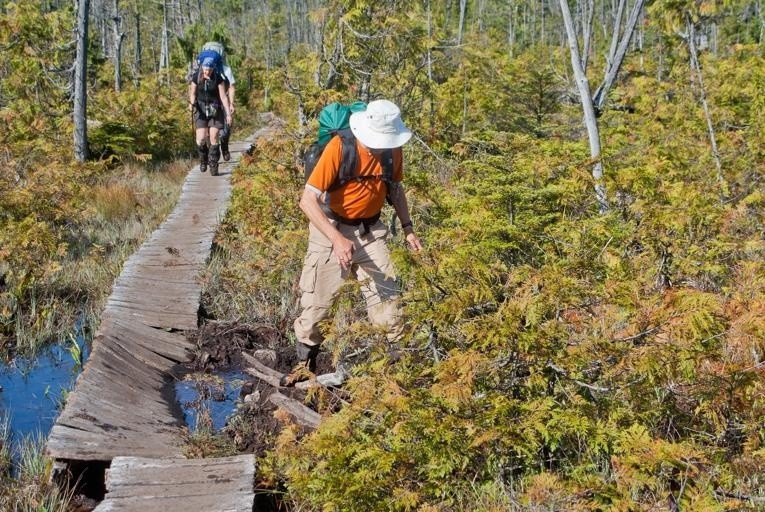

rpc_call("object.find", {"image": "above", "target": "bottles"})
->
[306,145,319,165]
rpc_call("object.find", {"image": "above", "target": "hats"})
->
[202,57,216,69]
[348,99,413,151]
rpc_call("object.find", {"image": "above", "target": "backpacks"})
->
[196,50,223,75]
[303,101,394,227]
[202,41,227,65]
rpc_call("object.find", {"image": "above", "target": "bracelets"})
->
[402,221,413,229]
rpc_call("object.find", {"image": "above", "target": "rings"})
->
[340,256,344,260]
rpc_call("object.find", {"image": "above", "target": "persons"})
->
[218,64,237,161]
[279,99,423,388]
[186,58,233,177]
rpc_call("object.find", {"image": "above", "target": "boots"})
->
[208,143,221,176]
[195,141,209,172]
[280,341,321,387]
[220,133,231,161]
